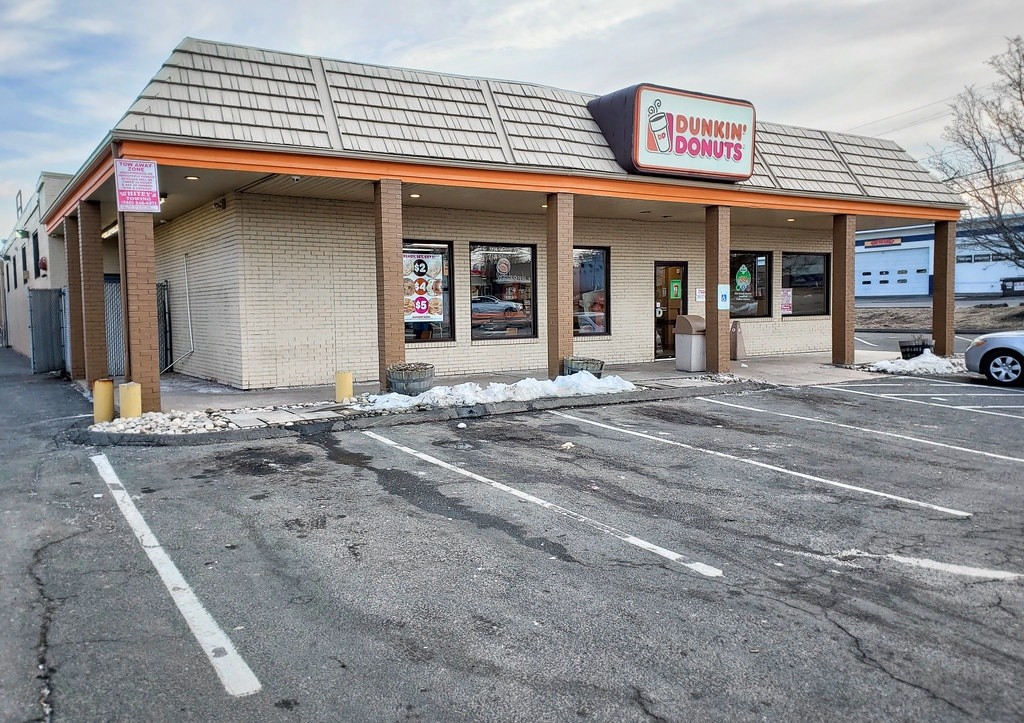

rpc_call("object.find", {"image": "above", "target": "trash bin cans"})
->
[672,315,707,372]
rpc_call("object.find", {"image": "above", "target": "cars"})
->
[963,330,1024,387]
[471,295,523,314]
